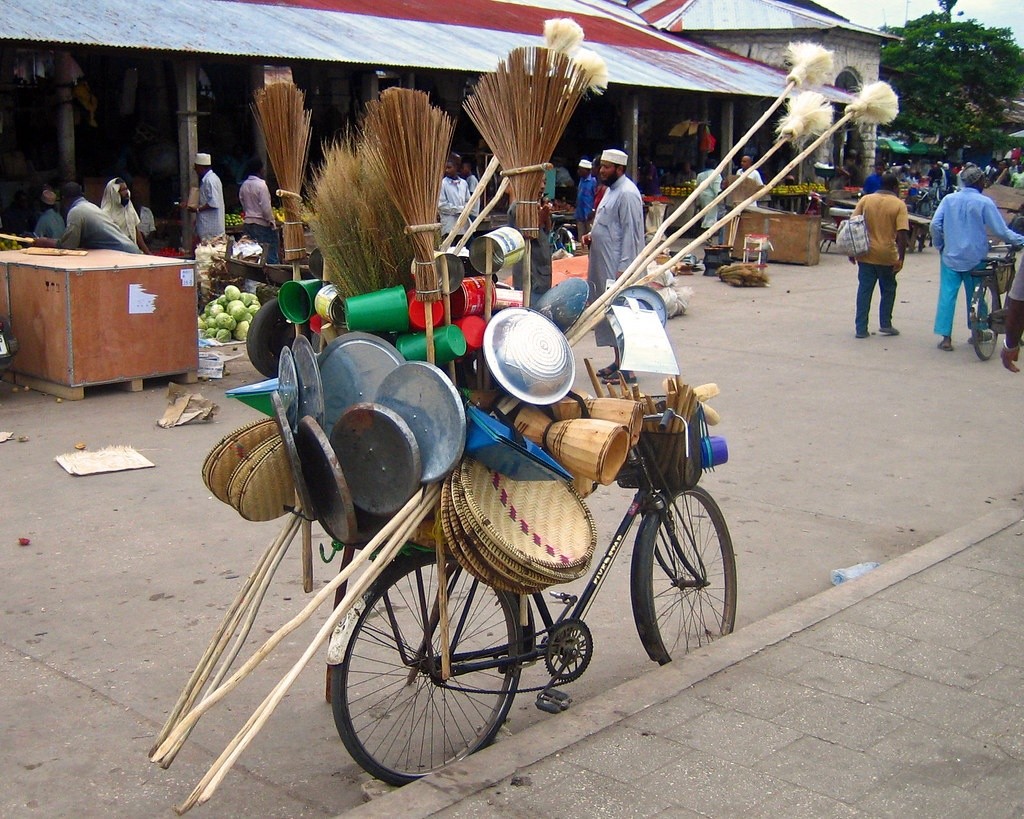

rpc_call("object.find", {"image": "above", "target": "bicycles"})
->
[907,183,935,214]
[324,378,738,788]
[968,239,1024,361]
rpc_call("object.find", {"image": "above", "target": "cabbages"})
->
[198,284,261,344]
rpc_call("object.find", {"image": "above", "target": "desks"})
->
[0,249,198,402]
[723,215,821,267]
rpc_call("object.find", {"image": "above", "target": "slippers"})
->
[597,363,619,376]
[967,330,992,343]
[602,370,636,384]
[938,341,953,351]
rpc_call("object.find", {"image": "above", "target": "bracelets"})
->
[197,207,200,213]
[1003,340,1019,351]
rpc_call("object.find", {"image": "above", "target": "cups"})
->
[278,278,322,323]
[343,284,485,361]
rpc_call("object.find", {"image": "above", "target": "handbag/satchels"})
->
[699,126,715,153]
[992,250,1012,294]
[836,213,869,256]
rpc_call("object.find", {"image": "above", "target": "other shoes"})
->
[880,326,899,335]
[856,331,869,338]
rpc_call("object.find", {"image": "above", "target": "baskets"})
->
[204,417,297,522]
[614,393,702,489]
[440,450,597,595]
[208,268,245,301]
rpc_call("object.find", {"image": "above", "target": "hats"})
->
[601,149,628,166]
[904,164,910,169]
[579,160,593,169]
[40,190,56,205]
[937,161,943,166]
[195,153,211,166]
[960,164,981,186]
[245,157,263,173]
[943,163,949,170]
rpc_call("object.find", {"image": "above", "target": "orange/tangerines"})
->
[0,238,23,251]
[769,182,827,194]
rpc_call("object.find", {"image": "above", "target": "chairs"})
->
[820,228,837,252]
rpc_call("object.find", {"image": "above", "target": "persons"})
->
[0,177,157,256]
[581,149,648,385]
[735,156,763,206]
[238,156,281,264]
[695,159,724,246]
[845,148,1024,247]
[929,166,1024,351]
[436,154,606,307]
[179,153,226,245]
[1001,254,1024,372]
[847,174,910,338]
[636,154,697,196]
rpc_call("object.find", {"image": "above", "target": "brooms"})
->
[305,131,410,703]
[250,81,314,593]
[460,46,592,628]
[360,86,455,681]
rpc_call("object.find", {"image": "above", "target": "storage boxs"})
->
[199,353,223,378]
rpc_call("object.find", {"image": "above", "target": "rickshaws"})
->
[981,168,1024,253]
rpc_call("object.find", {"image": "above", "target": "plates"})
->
[483,307,576,404]
